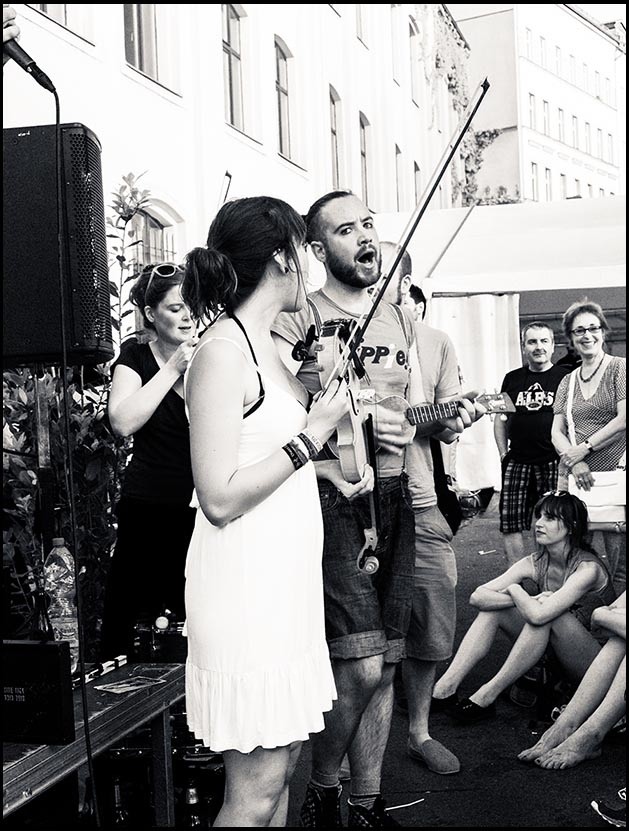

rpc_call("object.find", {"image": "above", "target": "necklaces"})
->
[580,351,606,383]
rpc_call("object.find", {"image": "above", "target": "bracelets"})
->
[292,437,309,460]
[297,433,319,459]
[281,444,303,471]
[301,429,324,453]
[288,441,308,466]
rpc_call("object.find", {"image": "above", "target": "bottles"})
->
[41,538,79,675]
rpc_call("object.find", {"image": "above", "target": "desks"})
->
[3,662,187,830]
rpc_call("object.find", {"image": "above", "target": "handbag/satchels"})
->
[568,470,626,533]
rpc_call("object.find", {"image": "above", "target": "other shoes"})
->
[407,735,460,774]
[299,783,344,827]
[598,785,627,822]
[396,692,459,714]
[449,698,497,720]
[347,796,401,826]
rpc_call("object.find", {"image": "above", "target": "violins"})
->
[337,387,379,575]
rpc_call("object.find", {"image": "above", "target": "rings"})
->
[578,478,582,482]
[566,461,569,465]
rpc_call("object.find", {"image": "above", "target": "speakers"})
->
[1,121,115,370]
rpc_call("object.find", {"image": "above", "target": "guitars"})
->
[327,387,516,459]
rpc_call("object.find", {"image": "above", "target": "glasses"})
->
[145,264,185,305]
[571,326,603,336]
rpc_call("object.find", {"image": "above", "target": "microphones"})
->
[3,24,57,95]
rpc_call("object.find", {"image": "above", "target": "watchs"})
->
[584,440,595,454]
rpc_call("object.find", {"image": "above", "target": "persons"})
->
[108,191,626,827]
